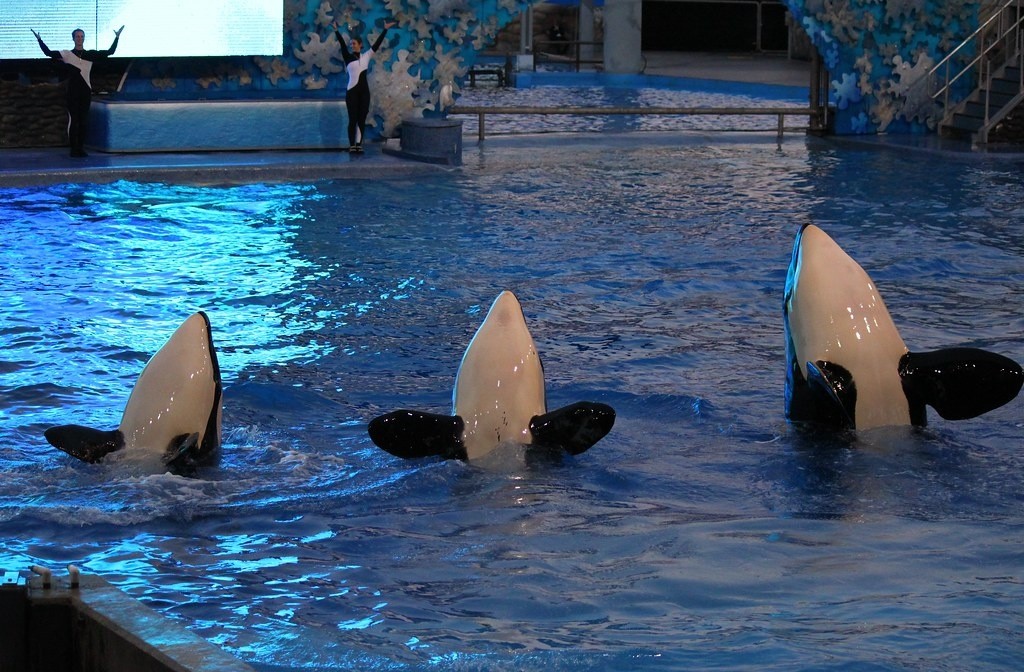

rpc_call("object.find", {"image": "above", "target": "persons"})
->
[330,20,396,152]
[31,26,124,158]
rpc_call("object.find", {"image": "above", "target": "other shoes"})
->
[70,150,88,159]
[349,146,363,155]
